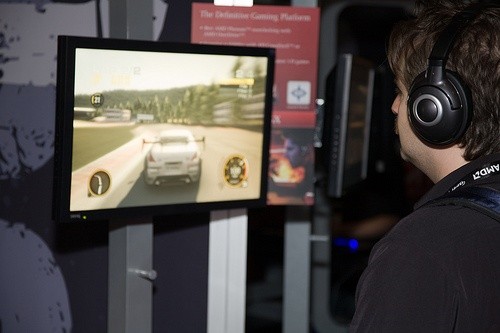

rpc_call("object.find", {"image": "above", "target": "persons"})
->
[345,0,500,333]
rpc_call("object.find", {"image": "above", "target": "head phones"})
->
[407,2,497,150]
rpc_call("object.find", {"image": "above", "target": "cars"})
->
[143,129,206,187]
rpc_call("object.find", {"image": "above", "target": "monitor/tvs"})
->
[322,55,378,199]
[54,35,277,223]
